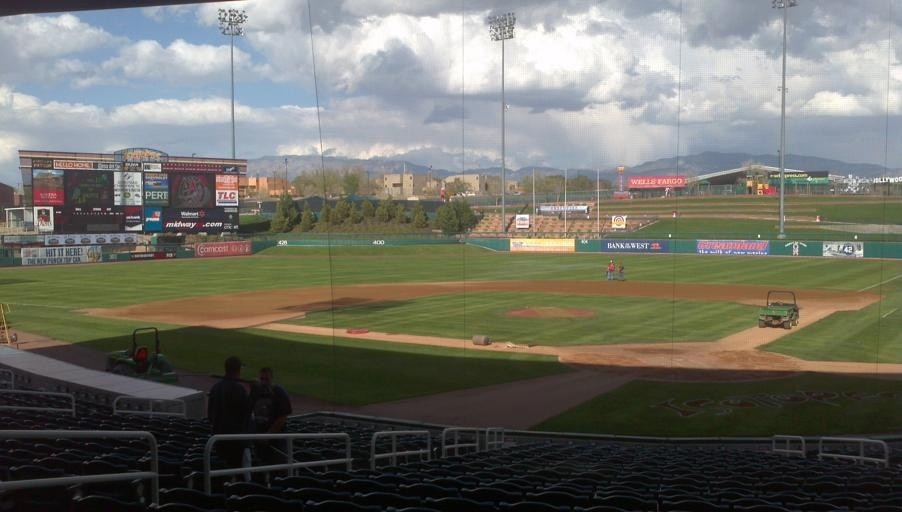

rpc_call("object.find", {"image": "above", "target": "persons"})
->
[617,262,625,282]
[208,357,254,482]
[607,259,616,281]
[40,208,49,221]
[250,366,293,486]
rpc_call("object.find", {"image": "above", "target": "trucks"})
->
[758,291,800,330]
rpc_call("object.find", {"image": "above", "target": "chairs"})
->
[1,382,902,507]
[468,213,649,239]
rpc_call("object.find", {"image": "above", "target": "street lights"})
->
[773,0,798,238]
[218,10,247,160]
[487,11,516,233]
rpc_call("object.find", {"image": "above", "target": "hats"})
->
[225,356,246,369]
